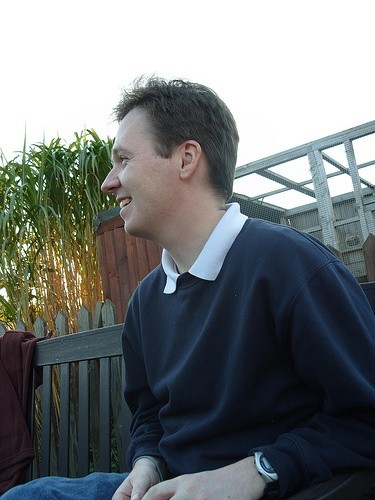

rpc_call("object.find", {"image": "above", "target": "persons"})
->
[0,73,374,500]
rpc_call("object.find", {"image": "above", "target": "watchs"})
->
[250,450,280,486]
